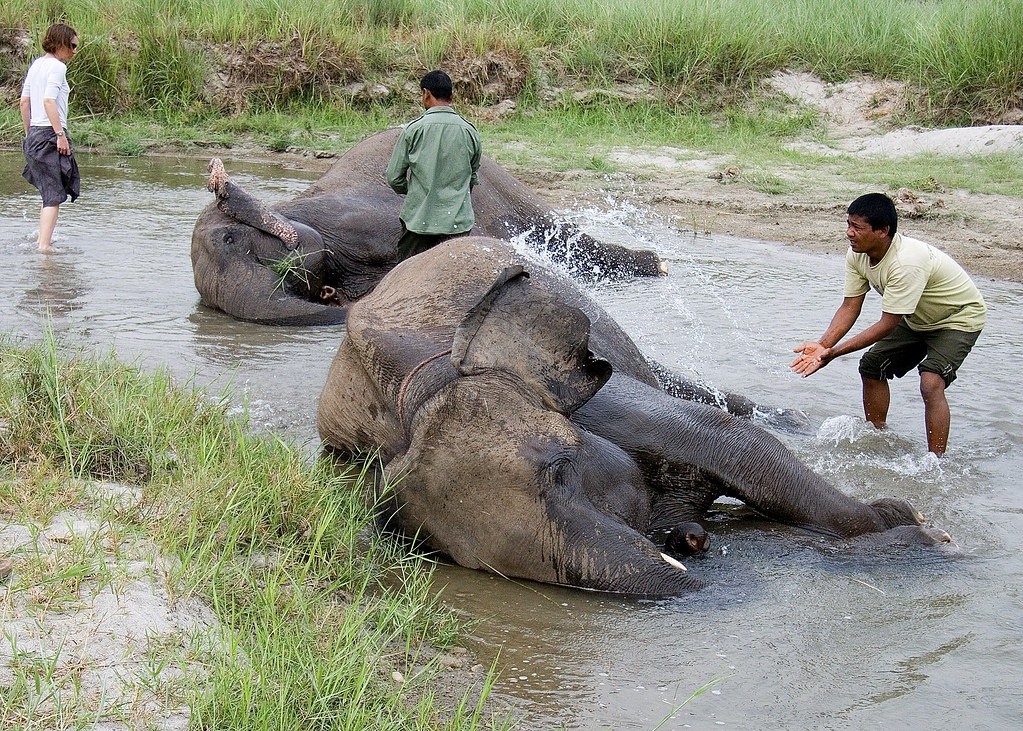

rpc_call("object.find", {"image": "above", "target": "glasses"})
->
[69,43,77,50]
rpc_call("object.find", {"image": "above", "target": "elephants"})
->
[190,124,960,606]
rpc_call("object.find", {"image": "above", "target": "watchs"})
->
[55,131,65,136]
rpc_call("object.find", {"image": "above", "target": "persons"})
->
[387,71,482,264]
[791,193,987,457]
[19,24,81,243]
